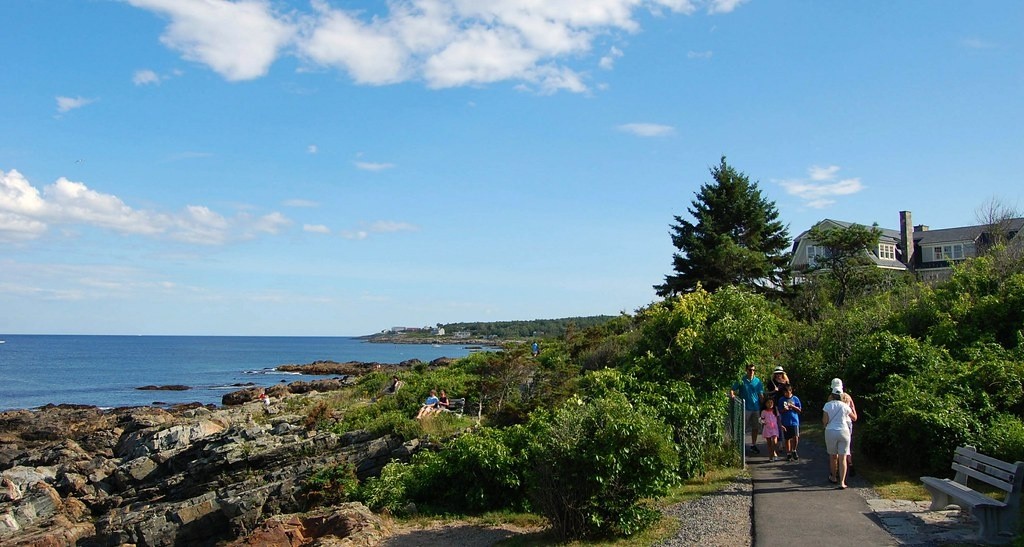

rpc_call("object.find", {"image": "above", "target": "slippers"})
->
[828,475,838,483]
[841,483,848,489]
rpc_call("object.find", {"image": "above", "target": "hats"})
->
[831,378,842,389]
[773,366,784,373]
[832,387,843,395]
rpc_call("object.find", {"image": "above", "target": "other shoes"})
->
[849,465,856,477]
[769,459,773,462]
[774,456,782,460]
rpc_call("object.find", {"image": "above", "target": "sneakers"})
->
[786,455,792,461]
[792,451,798,460]
[750,445,760,454]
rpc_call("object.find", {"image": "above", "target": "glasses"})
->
[746,369,756,372]
[775,373,782,375]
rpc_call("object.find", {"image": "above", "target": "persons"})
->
[370,375,399,402]
[730,364,765,451]
[765,365,791,449]
[778,384,802,462]
[531,341,538,357]
[427,390,449,417]
[761,397,787,462]
[417,389,439,420]
[822,387,857,489]
[827,378,857,477]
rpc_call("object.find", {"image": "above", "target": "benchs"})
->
[385,381,403,399]
[445,398,465,420]
[919,446,1024,545]
